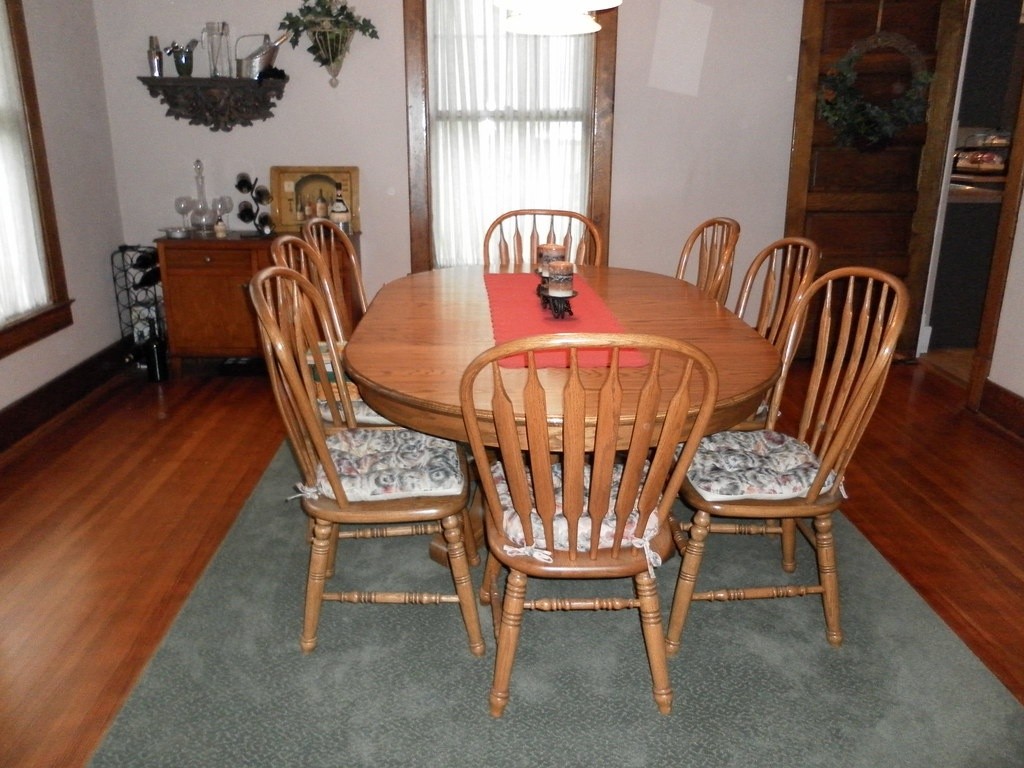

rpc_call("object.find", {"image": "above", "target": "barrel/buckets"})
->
[235,34,279,82]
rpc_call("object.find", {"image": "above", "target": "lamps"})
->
[235,172,272,237]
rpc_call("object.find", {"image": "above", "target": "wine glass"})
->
[176,196,233,237]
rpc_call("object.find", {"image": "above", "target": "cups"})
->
[173,50,193,78]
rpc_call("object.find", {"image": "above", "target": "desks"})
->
[340,260,782,573]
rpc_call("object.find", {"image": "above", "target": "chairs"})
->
[271,215,405,435]
[480,208,822,435]
[469,333,718,718]
[249,266,486,660]
[655,264,910,658]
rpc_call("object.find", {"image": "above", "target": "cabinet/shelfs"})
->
[150,227,362,383]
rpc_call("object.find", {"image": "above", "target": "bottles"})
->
[327,195,334,218]
[316,189,326,218]
[305,194,312,219]
[214,216,227,239]
[147,36,162,77]
[246,32,292,59]
[190,160,215,227]
[331,182,351,237]
[296,194,304,221]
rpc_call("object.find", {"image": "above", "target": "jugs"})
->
[201,21,233,78]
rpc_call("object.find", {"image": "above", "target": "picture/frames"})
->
[269,164,362,232]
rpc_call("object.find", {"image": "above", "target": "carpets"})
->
[85,435,1024,768]
